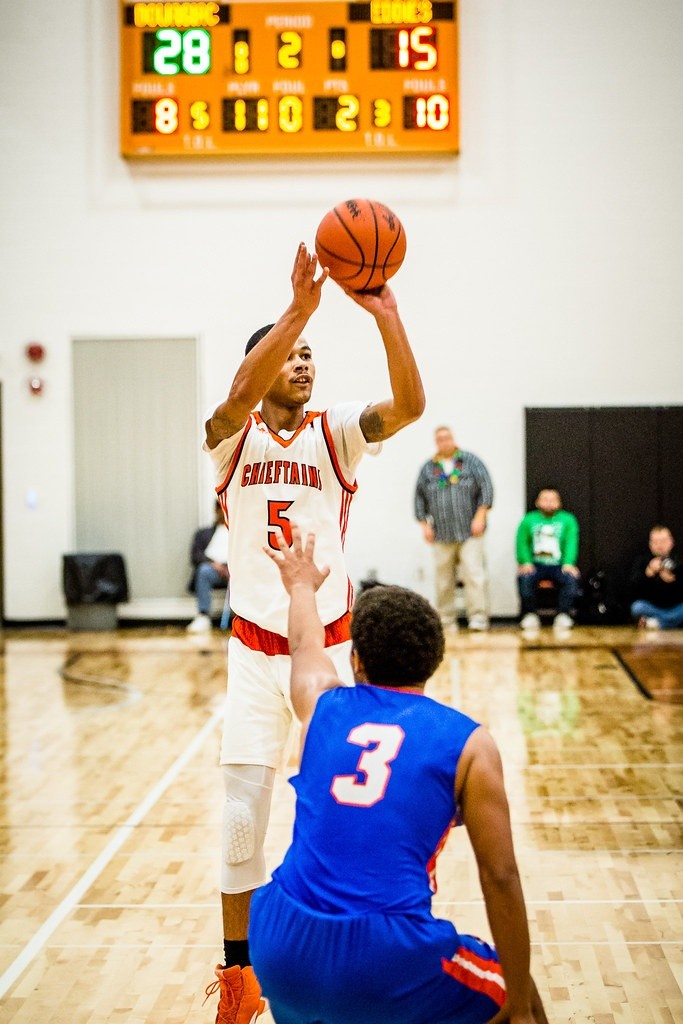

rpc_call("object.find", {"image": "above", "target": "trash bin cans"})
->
[60,554,129,633]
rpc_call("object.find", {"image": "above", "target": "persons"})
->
[412,426,492,632]
[204,242,428,1024]
[629,523,682,630]
[516,485,582,632]
[246,523,552,1024]
[187,497,236,634]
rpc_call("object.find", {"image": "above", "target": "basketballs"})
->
[314,198,406,291]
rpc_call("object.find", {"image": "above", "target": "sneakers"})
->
[186,614,212,631]
[636,614,647,631]
[554,612,574,629]
[519,612,541,629]
[468,616,490,631]
[202,963,269,1024]
[441,622,459,632]
